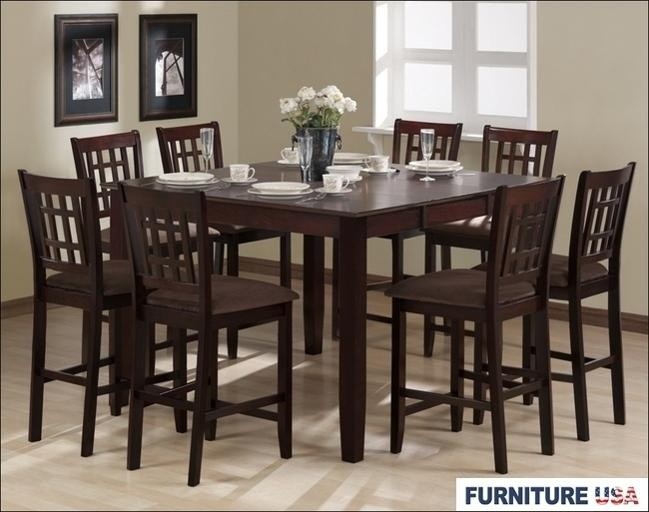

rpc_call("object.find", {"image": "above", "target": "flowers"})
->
[279,83,360,129]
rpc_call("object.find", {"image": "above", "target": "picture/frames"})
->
[139,12,200,122]
[53,11,119,129]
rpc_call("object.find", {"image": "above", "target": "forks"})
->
[291,191,331,207]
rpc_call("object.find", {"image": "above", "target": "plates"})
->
[156,179,218,185]
[198,125,466,201]
[159,172,215,181]
[166,186,208,189]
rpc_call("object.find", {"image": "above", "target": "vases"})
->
[292,127,344,182]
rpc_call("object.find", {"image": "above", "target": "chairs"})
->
[384,172,565,476]
[421,124,558,358]
[15,167,190,460]
[154,121,291,359]
[471,160,638,443]
[67,130,224,355]
[330,117,463,350]
[117,181,307,489]
